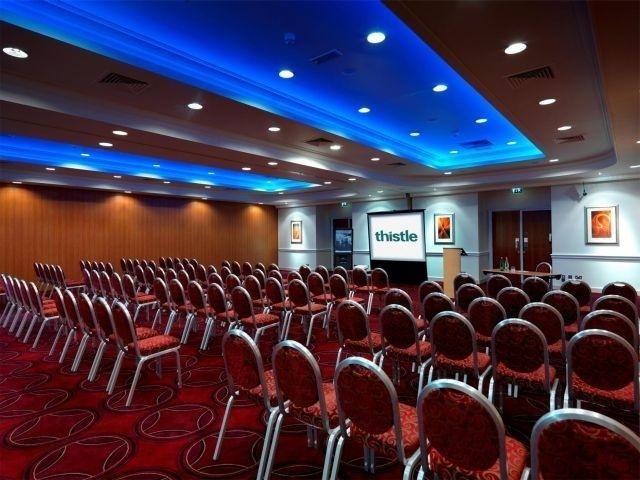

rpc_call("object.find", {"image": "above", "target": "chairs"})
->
[455,283,486,318]
[559,279,592,313]
[428,311,494,397]
[416,379,531,479]
[467,297,507,356]
[212,330,294,479]
[328,357,438,480]
[518,301,570,372]
[602,281,637,303]
[542,289,579,333]
[567,329,639,418]
[521,277,549,303]
[591,296,640,327]
[1,259,221,406]
[497,286,531,319]
[378,305,437,416]
[487,275,512,297]
[384,287,429,373]
[531,407,639,480]
[454,272,476,293]
[261,341,371,479]
[535,262,551,273]
[489,318,567,441]
[421,293,457,343]
[417,280,455,320]
[334,303,399,394]
[331,266,390,315]
[222,261,331,341]
[579,310,640,351]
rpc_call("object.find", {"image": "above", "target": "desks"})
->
[483,269,562,287]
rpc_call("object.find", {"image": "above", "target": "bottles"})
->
[504,256,510,273]
[500,256,504,272]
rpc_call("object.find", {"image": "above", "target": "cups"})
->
[510,266,516,273]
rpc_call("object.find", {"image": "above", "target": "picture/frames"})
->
[433,213,455,245]
[291,220,303,244]
[585,204,619,245]
